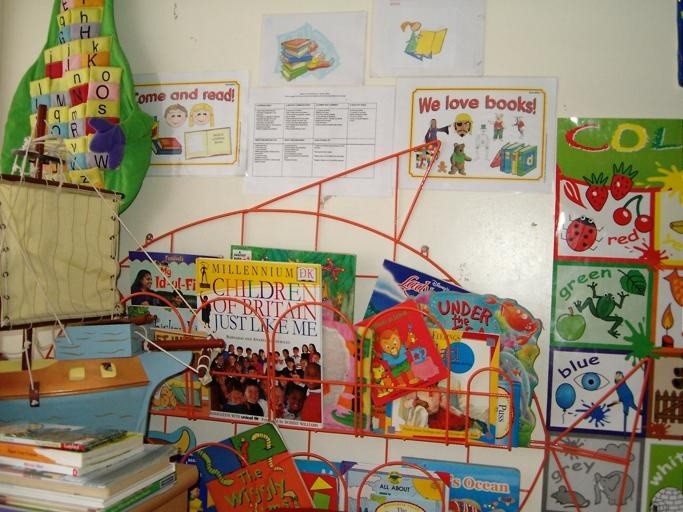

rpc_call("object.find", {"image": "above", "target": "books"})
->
[415,26,448,56]
[280,37,334,81]
[152,137,182,155]
[491,142,538,176]
[0,417,177,512]
[184,127,232,160]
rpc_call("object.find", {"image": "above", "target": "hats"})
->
[453,113,473,124]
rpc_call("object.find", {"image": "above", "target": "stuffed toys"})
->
[448,143,471,175]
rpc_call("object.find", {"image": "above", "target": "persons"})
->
[404,382,473,430]
[216,355,248,398]
[283,387,303,420]
[223,347,228,359]
[265,352,272,374]
[275,351,287,375]
[224,380,246,413]
[242,352,249,373]
[260,379,268,397]
[275,357,289,386]
[314,352,320,362]
[301,363,321,422]
[283,358,296,375]
[235,362,243,373]
[246,348,252,361]
[242,385,263,416]
[228,344,235,355]
[250,353,263,374]
[293,369,307,397]
[239,356,244,365]
[237,346,243,361]
[211,353,226,405]
[264,386,284,418]
[141,300,149,306]
[302,344,308,353]
[300,353,313,370]
[169,289,192,308]
[283,349,291,364]
[246,365,257,385]
[291,346,302,370]
[258,349,265,364]
[131,270,160,306]
[308,344,316,362]
[199,290,211,329]
[279,374,297,401]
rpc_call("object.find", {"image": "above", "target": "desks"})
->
[2,463,199,512]
